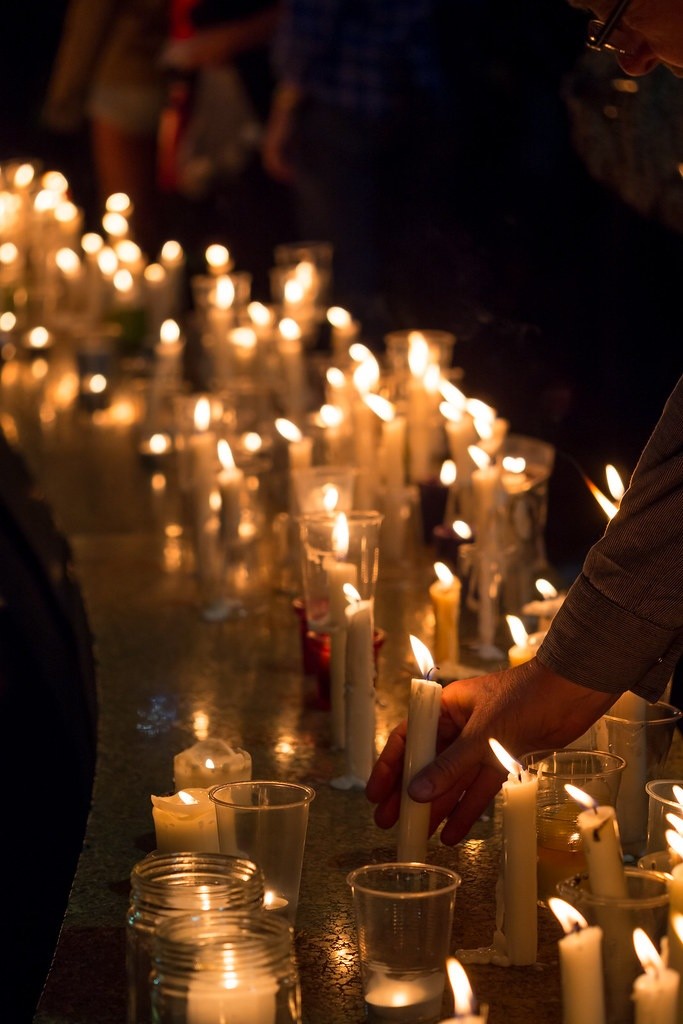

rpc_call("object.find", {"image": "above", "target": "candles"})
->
[0,156,683,1024]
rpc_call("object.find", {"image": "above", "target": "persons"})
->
[365,0,683,847]
[43,0,683,568]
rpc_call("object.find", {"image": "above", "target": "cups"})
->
[128,854,265,1024]
[151,910,303,1024]
[0,168,552,710]
[589,701,683,847]
[519,750,627,906]
[347,863,460,1024]
[640,852,683,969]
[211,780,315,934]
[645,780,683,850]
[559,870,669,1024]
